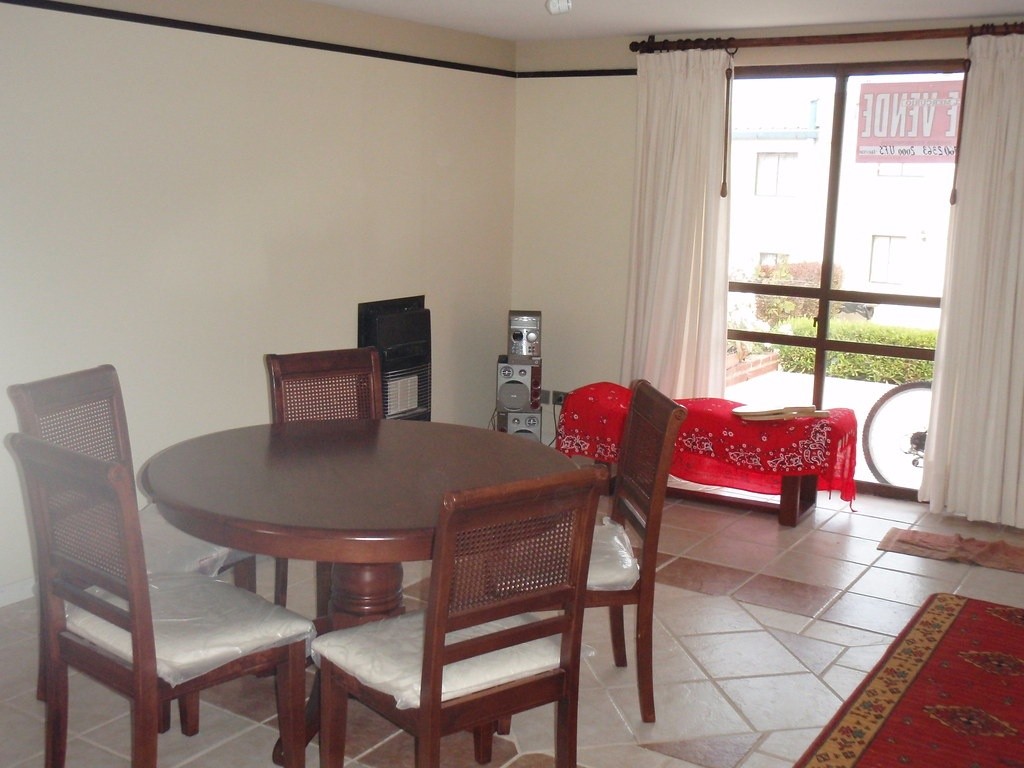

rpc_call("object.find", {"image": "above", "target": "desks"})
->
[136,416,585,761]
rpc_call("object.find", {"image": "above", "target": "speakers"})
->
[496,355,542,443]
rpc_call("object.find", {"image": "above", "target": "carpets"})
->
[781,594,1024,768]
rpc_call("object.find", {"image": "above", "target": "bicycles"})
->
[862,379,934,487]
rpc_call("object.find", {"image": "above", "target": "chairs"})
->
[495,378,687,723]
[8,364,258,733]
[265,346,385,607]
[311,467,610,768]
[12,434,318,768]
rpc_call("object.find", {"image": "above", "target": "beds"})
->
[556,382,858,526]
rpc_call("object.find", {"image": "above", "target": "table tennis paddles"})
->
[731,403,832,421]
